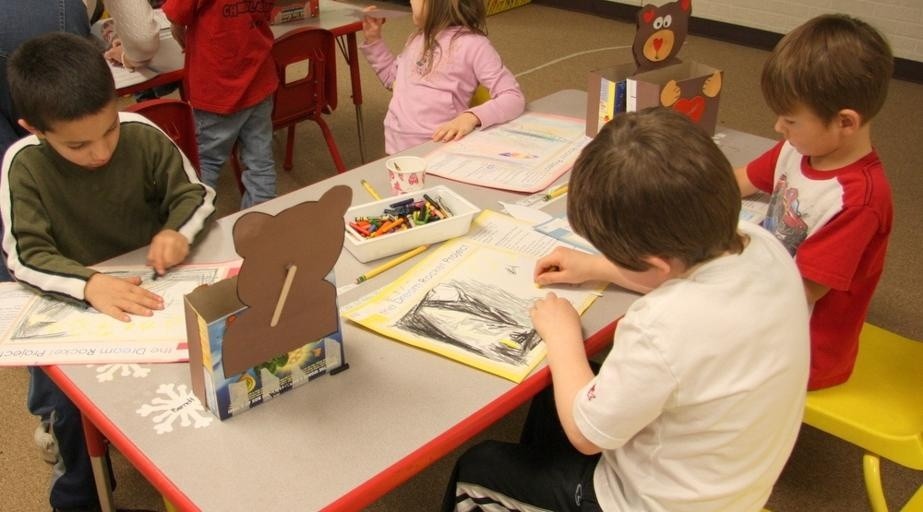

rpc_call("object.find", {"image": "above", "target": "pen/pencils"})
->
[353,244,434,284]
[360,178,381,201]
[341,194,454,239]
[543,185,570,201]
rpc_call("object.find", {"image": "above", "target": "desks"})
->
[38,89,780,512]
[99,0,386,164]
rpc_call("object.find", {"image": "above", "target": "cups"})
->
[385,157,424,193]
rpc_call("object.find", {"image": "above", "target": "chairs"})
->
[265,27,346,175]
[122,99,200,180]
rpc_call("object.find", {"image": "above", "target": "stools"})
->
[802,322,923,512]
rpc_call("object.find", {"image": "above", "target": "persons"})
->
[444,108,812,511]
[0,0,160,279]
[361,1,526,160]
[0,31,217,512]
[729,12,893,395]
[161,2,279,218]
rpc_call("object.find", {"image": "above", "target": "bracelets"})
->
[122,53,126,68]
